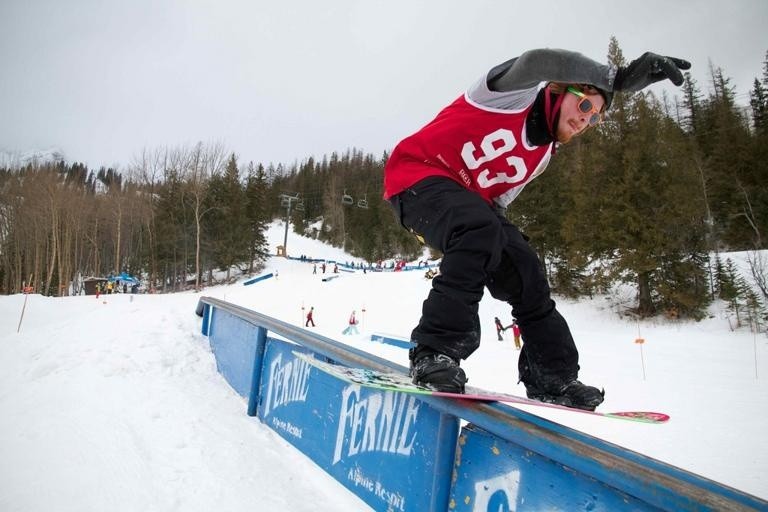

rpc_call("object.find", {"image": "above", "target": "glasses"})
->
[568,85,601,127]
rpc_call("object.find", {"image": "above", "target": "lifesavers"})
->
[23,286,33,293]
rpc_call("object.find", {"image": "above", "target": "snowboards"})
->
[343,320,360,334]
[292,348,670,425]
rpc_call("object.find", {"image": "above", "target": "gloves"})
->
[612,51,691,94]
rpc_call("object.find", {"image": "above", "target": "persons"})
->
[95,280,129,299]
[348,310,359,336]
[300,254,408,275]
[305,307,315,328]
[383,46,691,412]
[494,315,505,341]
[416,259,438,280]
[504,318,522,350]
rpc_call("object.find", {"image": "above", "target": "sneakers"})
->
[408,351,466,387]
[526,376,604,406]
